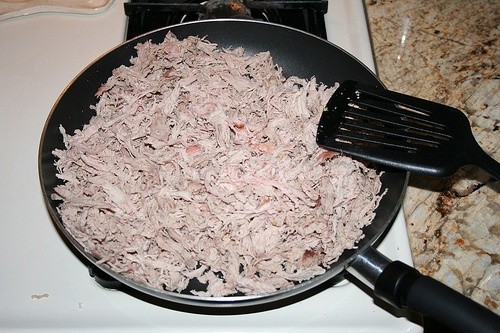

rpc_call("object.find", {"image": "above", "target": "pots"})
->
[37,18,500,333]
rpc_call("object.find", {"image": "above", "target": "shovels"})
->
[316,84,500,196]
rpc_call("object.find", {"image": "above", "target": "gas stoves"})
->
[88,0,424,333]
